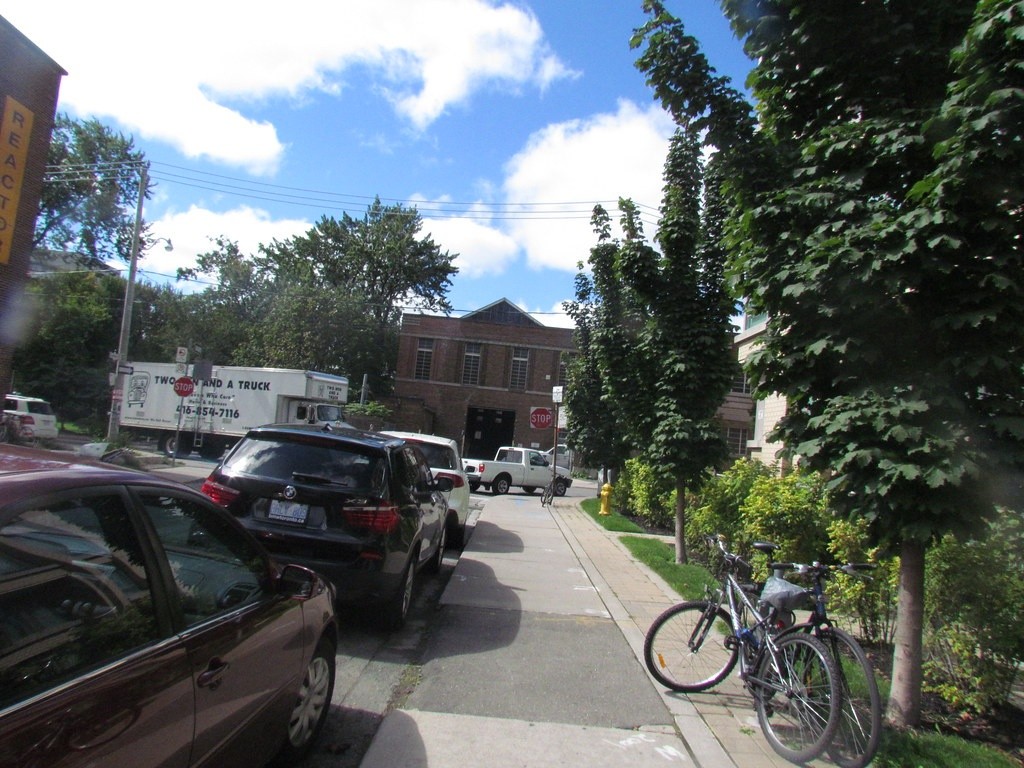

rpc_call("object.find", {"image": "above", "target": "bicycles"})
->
[1,411,36,446]
[643,533,841,765]
[734,540,884,767]
[540,468,564,508]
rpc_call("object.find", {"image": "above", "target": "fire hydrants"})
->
[598,483,614,517]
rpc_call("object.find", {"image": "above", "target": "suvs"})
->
[1,394,60,443]
[351,428,471,547]
[185,421,451,637]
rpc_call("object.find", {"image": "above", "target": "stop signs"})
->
[530,407,553,429]
[173,376,195,397]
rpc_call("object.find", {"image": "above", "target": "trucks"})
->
[117,362,352,461]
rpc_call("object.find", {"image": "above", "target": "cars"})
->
[0,437,342,768]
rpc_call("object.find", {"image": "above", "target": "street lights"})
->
[106,238,175,444]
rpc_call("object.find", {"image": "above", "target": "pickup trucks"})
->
[459,447,575,496]
